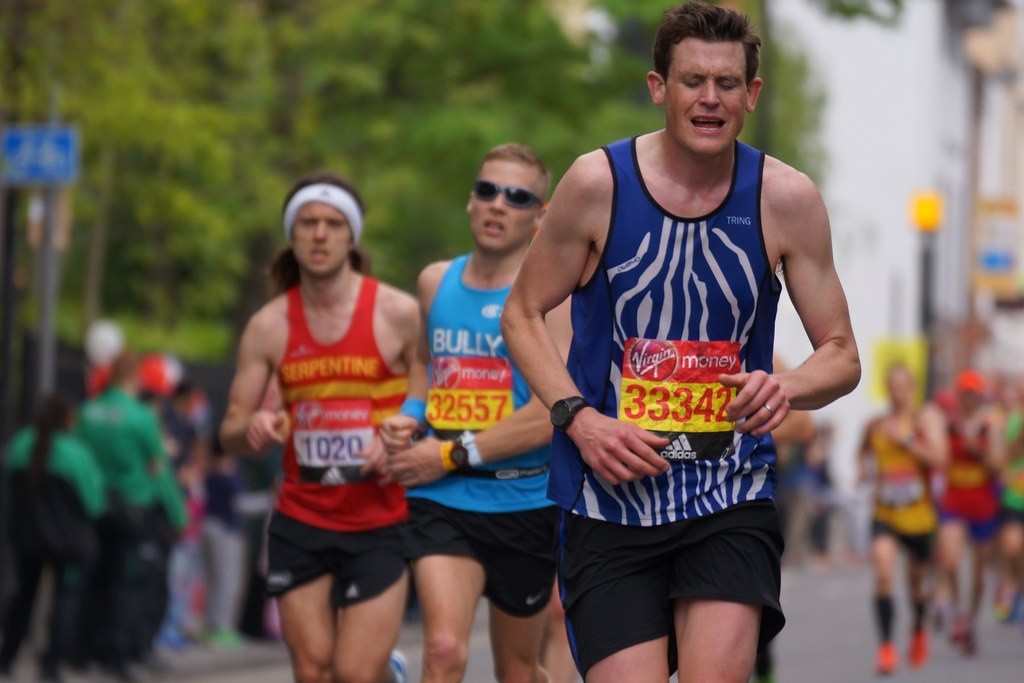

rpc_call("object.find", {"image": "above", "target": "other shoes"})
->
[154,628,185,653]
[390,652,407,682]
[206,628,241,649]
[879,644,898,673]
[909,629,931,667]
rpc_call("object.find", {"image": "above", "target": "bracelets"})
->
[440,440,456,471]
[460,430,484,468]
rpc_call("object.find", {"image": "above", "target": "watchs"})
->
[451,439,469,471]
[548,395,592,433]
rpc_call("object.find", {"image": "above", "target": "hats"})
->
[140,353,182,400]
[959,370,982,395]
[83,320,123,366]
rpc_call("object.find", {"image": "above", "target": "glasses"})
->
[473,179,543,208]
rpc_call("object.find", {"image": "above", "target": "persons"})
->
[213,177,420,683]
[500,4,861,683]
[0,354,284,683]
[927,369,1002,653]
[377,143,571,683]
[855,362,949,673]
[929,379,1024,629]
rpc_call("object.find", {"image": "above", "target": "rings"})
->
[764,403,774,414]
